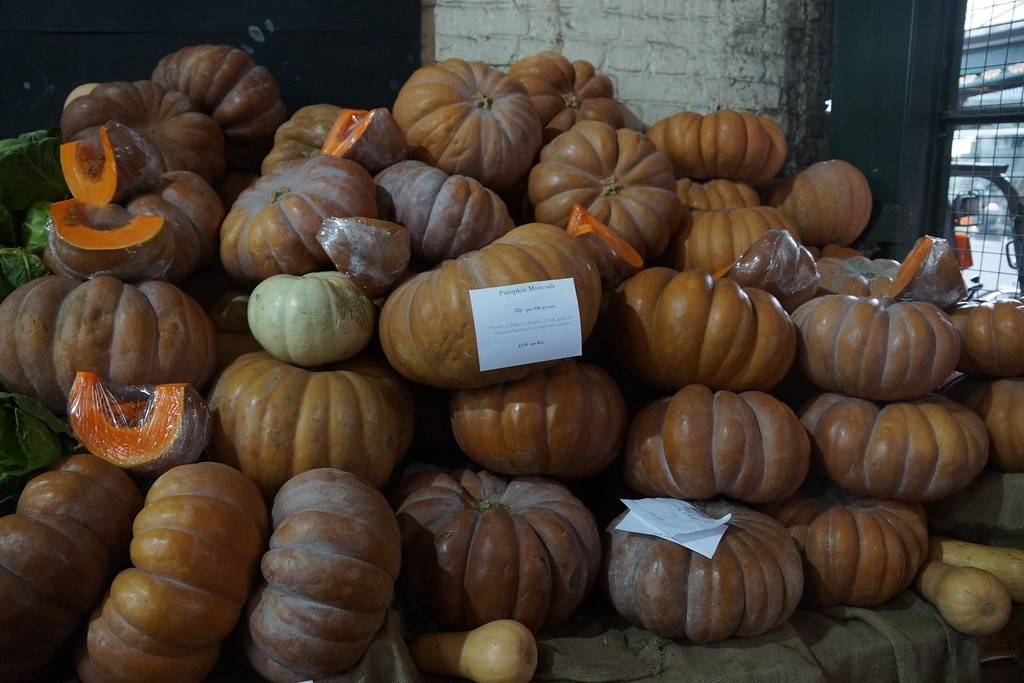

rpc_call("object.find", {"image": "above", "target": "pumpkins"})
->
[0,44,1024,683]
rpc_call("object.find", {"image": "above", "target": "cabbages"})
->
[0,130,64,505]
